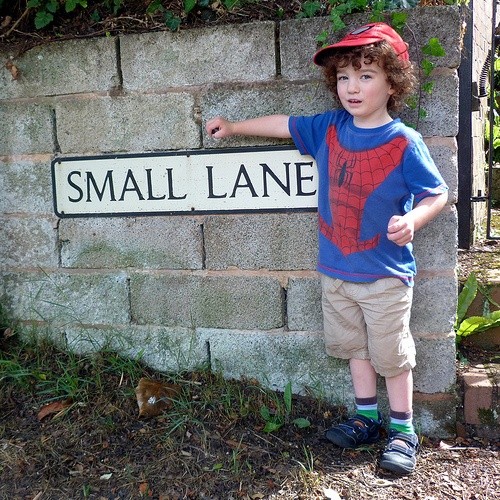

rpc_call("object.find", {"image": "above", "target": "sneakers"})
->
[325,413,386,449]
[380,426,426,474]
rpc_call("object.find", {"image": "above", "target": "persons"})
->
[205,23,449,473]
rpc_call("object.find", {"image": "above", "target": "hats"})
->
[312,21,414,74]
